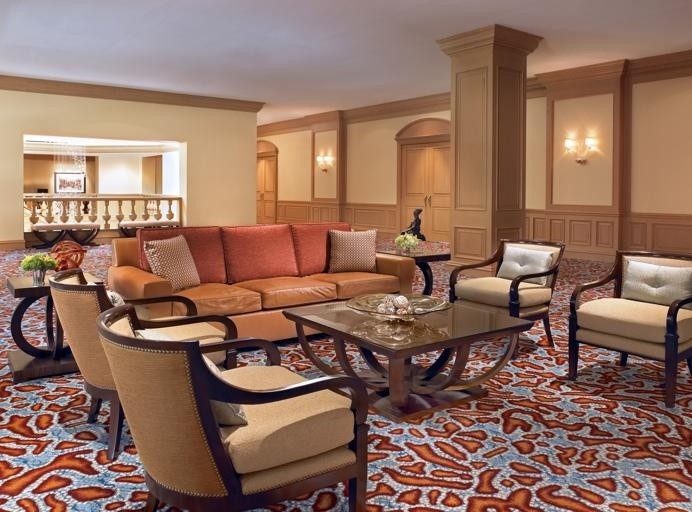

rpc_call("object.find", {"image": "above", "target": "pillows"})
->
[497,242,553,286]
[136,227,227,284]
[87,280,125,308]
[220,223,300,284]
[134,327,248,428]
[327,228,379,274]
[143,233,200,292]
[290,222,351,277]
[621,261,692,309]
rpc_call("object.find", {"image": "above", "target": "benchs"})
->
[30,224,103,249]
[119,220,182,238]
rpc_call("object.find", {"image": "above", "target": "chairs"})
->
[96,304,370,512]
[48,267,237,462]
[568,248,692,408]
[449,239,565,357]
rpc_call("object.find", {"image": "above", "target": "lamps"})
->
[316,155,333,172]
[565,138,595,165]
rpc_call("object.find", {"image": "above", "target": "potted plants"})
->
[21,252,57,285]
[395,232,421,254]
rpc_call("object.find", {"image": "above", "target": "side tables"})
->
[377,249,452,294]
[6,271,106,385]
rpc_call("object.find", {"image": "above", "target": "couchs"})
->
[107,222,417,350]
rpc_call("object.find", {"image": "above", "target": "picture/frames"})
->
[54,171,86,194]
